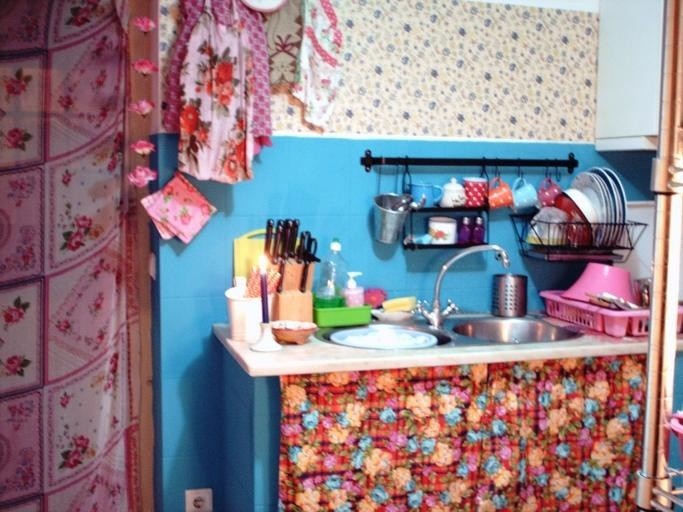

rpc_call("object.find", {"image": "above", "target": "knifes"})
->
[263,219,316,294]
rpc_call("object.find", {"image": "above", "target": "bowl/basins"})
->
[554,194,592,246]
[429,217,457,244]
[560,262,635,311]
[562,189,597,232]
[329,328,440,350]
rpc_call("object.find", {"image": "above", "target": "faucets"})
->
[430,240,513,331]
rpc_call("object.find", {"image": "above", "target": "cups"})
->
[488,177,512,210]
[539,176,561,206]
[441,176,466,206]
[512,178,538,211]
[410,181,443,208]
[464,176,487,205]
[370,193,408,245]
[224,280,273,340]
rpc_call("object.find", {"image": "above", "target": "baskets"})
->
[508,213,648,263]
[538,289,683,337]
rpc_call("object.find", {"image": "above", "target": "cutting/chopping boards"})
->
[231,229,262,279]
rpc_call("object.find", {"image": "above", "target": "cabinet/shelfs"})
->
[399,205,488,249]
[592,1,683,155]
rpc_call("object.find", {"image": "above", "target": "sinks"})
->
[311,321,455,354]
[443,316,587,348]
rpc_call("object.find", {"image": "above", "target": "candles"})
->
[257,257,269,322]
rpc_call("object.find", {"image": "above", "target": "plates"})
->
[372,308,412,322]
[571,166,624,188]
[587,189,627,206]
[597,206,627,246]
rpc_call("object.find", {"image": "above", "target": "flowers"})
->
[121,13,160,191]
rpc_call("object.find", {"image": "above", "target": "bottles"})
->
[473,215,485,244]
[459,215,471,243]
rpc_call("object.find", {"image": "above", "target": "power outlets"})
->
[184,488,213,512]
